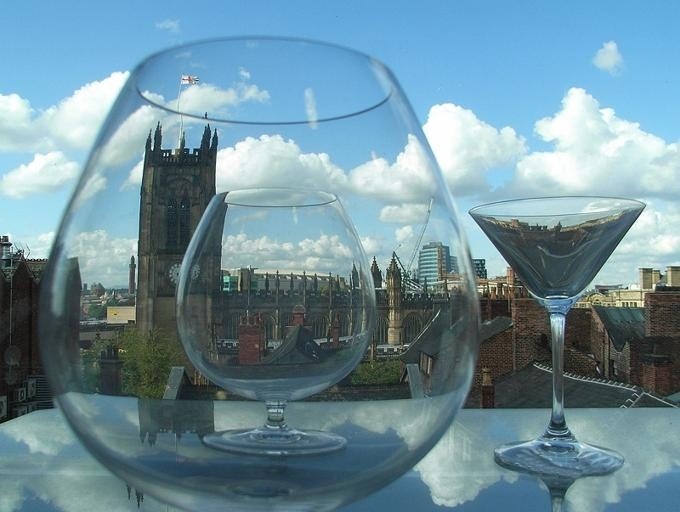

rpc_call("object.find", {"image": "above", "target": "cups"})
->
[33,33,483,512]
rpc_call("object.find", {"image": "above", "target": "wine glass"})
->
[174,186,382,459]
[468,195,648,481]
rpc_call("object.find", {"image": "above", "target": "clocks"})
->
[165,261,193,288]
[190,263,201,280]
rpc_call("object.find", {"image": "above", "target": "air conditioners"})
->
[0,395,7,418]
[14,388,27,402]
[12,406,27,418]
[23,379,36,398]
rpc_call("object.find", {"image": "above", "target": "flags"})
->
[181,75,201,85]
